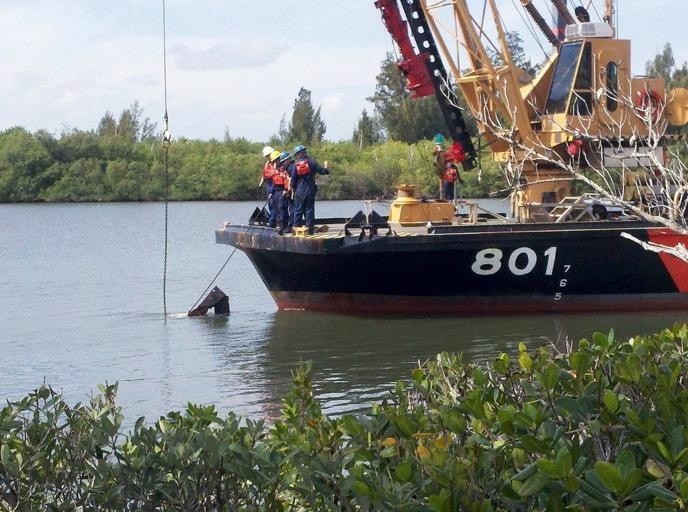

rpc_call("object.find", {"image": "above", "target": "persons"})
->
[259,146,295,232]
[290,145,329,234]
[443,162,463,200]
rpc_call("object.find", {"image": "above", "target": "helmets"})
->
[278,151,292,163]
[293,145,308,155]
[269,151,281,162]
[262,146,275,159]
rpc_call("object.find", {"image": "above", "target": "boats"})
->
[205,1,686,316]
